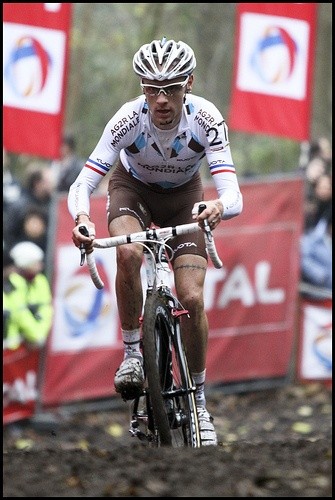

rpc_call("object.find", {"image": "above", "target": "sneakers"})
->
[114,353,144,388]
[191,407,218,446]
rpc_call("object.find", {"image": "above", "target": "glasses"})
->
[140,75,190,96]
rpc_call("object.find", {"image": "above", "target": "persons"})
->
[298,138,332,306]
[67,38,244,449]
[3,136,79,452]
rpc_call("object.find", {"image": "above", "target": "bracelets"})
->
[75,212,91,226]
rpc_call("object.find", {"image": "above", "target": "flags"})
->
[39,181,304,406]
[3,2,72,159]
[295,296,332,383]
[226,3,317,141]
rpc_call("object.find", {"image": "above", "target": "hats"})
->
[10,242,42,266]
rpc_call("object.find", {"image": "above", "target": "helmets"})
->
[133,37,196,80]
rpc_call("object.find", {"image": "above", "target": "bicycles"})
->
[79,202,225,450]
